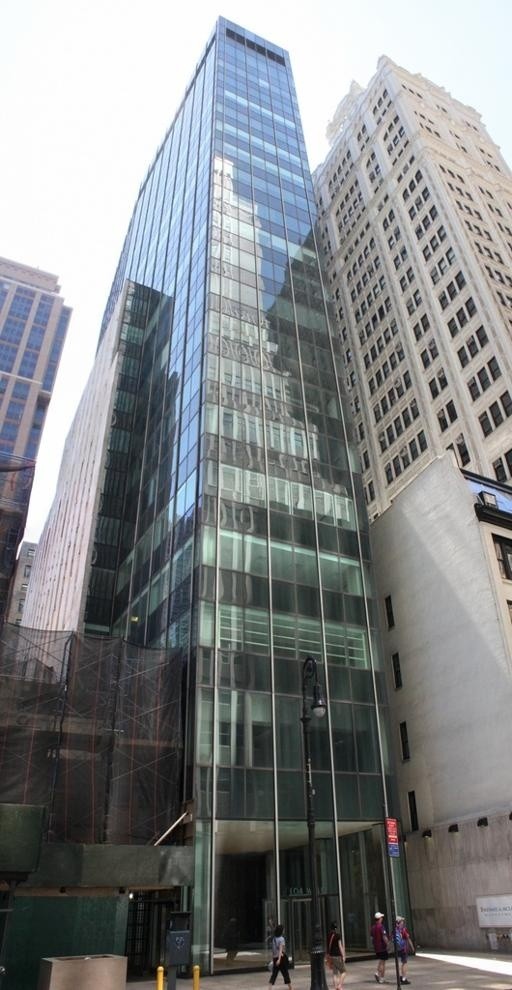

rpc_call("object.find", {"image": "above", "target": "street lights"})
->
[300,656,327,950]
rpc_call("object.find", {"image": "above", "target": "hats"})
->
[396,915,405,922]
[375,912,384,919]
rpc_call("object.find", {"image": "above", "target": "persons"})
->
[223,918,242,961]
[369,912,390,984]
[391,915,415,984]
[266,924,293,990]
[327,920,347,990]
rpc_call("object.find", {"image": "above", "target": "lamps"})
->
[448,824,458,833]
[423,829,432,839]
[398,835,406,844]
[477,817,488,827]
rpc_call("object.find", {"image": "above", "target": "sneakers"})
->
[400,976,410,985]
[375,973,385,983]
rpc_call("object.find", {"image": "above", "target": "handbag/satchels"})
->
[326,956,334,968]
[395,931,405,950]
[281,953,289,963]
